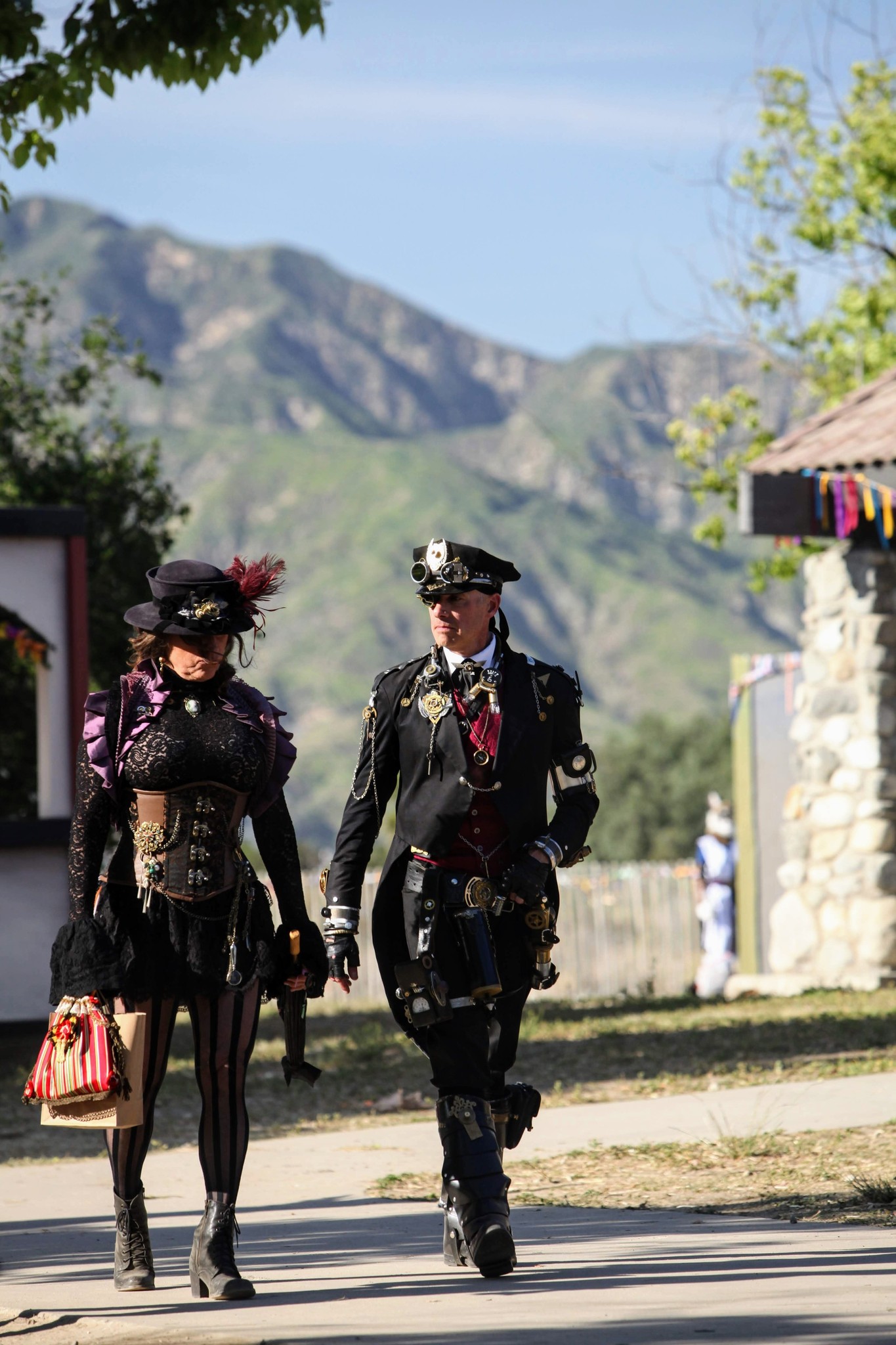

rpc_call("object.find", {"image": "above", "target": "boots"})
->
[437,1085,541,1278]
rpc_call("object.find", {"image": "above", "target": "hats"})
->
[124,559,255,635]
[413,538,521,594]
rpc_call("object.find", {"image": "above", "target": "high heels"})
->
[113,1185,156,1291]
[188,1199,256,1300]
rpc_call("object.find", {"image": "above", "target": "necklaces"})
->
[457,699,490,766]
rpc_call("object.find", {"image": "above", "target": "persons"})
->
[47,558,326,1299]
[690,788,735,998]
[316,537,600,1276]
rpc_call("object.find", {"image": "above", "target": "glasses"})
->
[411,562,474,583]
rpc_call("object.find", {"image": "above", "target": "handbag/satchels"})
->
[21,990,147,1130]
